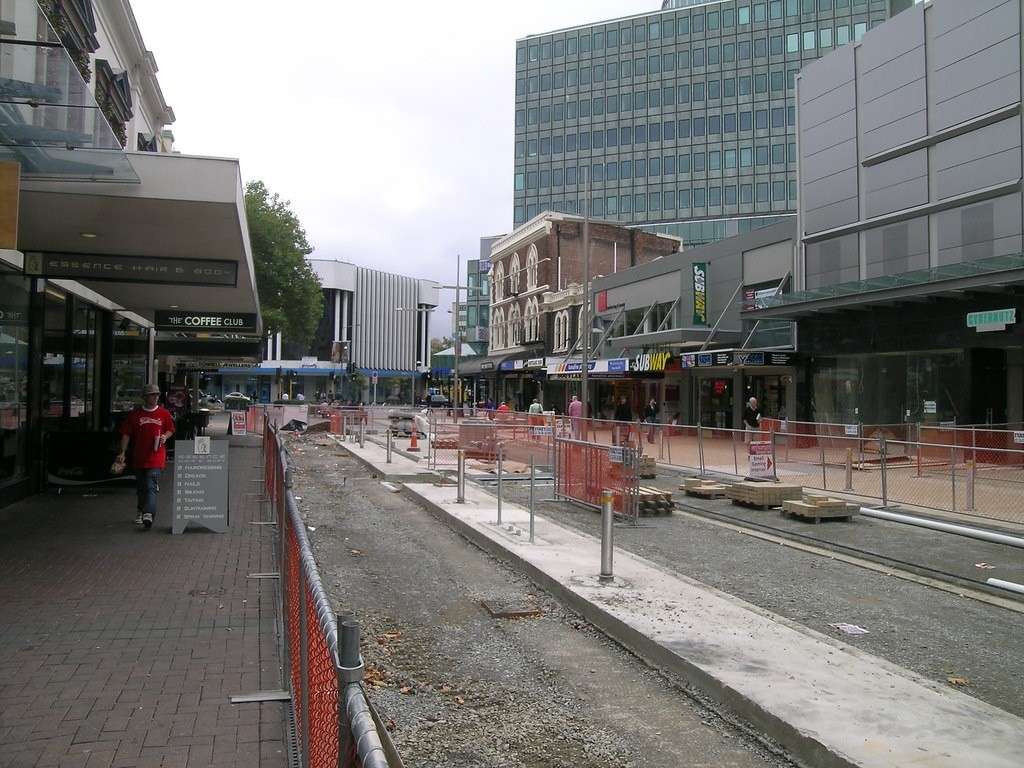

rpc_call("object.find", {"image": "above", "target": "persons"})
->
[115,384,175,528]
[313,390,333,400]
[415,392,421,404]
[550,402,558,415]
[297,392,304,400]
[529,399,543,439]
[743,397,762,461]
[278,392,289,400]
[321,399,360,413]
[569,395,583,440]
[645,399,658,443]
[485,397,496,420]
[497,402,509,419]
[426,395,433,416]
[614,394,632,442]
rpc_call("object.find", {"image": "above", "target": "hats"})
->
[142,384,161,396]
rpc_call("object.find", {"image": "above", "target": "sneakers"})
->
[135,512,143,524]
[143,513,152,528]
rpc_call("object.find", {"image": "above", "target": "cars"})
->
[224,392,251,402]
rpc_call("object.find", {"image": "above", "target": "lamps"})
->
[111,318,132,330]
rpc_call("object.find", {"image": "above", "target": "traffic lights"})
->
[275,378,279,385]
[292,371,297,378]
[286,370,291,378]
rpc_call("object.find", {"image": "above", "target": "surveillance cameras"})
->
[344,346,347,350]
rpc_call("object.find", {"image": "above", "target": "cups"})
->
[153,436,162,452]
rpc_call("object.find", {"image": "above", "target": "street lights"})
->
[432,254,484,423]
[394,307,436,407]
[545,164,627,442]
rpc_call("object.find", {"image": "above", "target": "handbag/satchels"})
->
[111,461,126,473]
[539,405,542,413]
